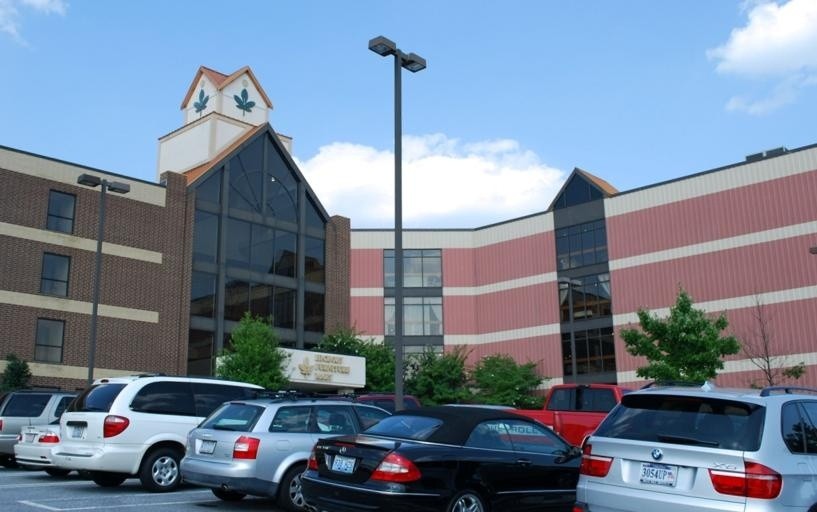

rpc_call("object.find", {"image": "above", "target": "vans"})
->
[324,393,422,426]
[50,374,266,493]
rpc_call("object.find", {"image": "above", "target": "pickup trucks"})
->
[501,382,637,478]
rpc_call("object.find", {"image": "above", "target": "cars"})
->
[571,378,817,512]
[12,418,93,480]
[299,406,585,512]
[178,391,393,512]
[0,385,85,470]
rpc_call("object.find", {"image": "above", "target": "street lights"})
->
[365,33,428,413]
[75,171,132,394]
